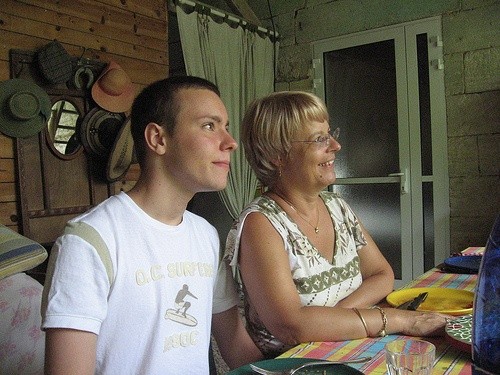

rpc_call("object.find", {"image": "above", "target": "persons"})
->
[41,76,264,375]
[221,91,456,358]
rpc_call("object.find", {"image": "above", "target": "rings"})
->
[445,318,448,323]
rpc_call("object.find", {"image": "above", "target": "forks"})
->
[249,356,372,375]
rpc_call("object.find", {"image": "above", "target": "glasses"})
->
[285,127,340,150]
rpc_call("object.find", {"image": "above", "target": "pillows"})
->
[0,224,48,280]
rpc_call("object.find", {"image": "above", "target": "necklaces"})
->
[285,201,319,233]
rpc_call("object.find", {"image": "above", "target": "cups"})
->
[384,339,435,375]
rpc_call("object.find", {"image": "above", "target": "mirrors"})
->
[44,96,85,160]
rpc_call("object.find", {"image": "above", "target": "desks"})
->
[274,247,485,375]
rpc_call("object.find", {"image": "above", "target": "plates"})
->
[444,255,482,273]
[386,287,475,316]
[225,358,365,375]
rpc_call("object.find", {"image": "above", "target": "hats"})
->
[92,62,134,113]
[0,79,51,138]
[106,116,134,182]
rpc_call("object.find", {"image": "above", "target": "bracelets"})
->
[352,308,369,336]
[371,306,387,337]
